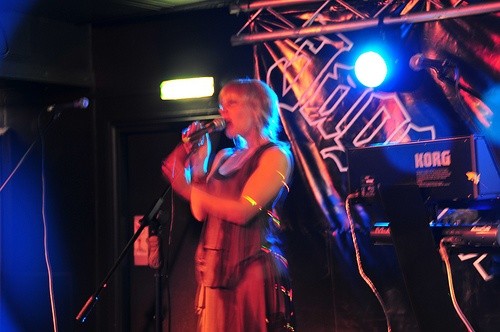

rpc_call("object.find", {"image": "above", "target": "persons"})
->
[160,80,293,332]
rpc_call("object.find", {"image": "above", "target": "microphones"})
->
[47,97,89,112]
[409,53,458,71]
[183,118,226,143]
